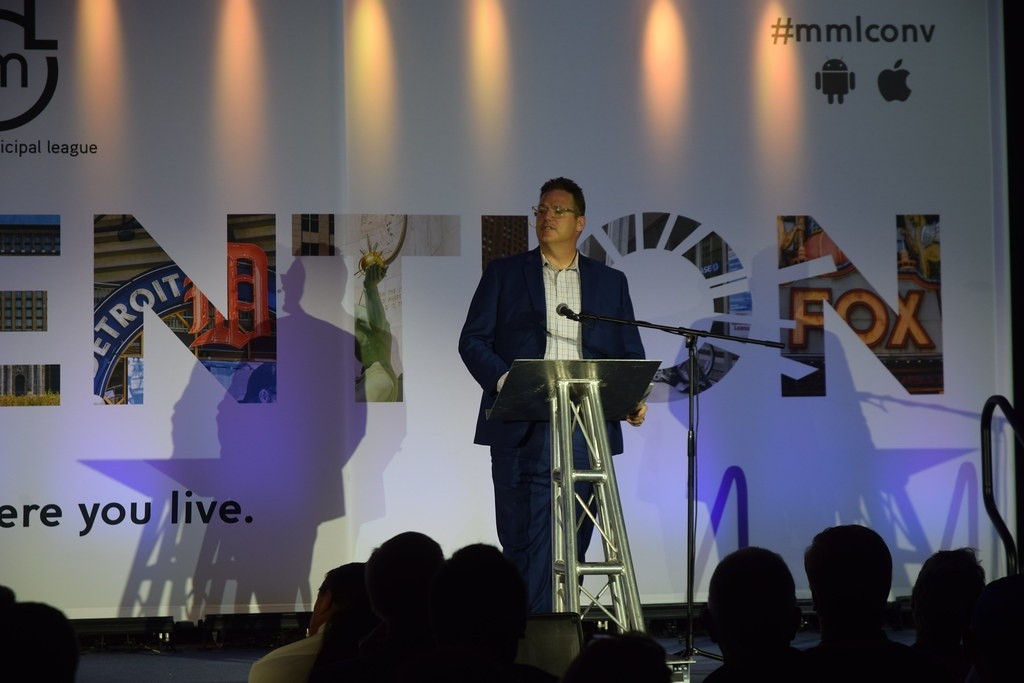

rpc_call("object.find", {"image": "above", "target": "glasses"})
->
[532,205,580,219]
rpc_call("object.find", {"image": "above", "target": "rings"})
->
[643,418,645,421]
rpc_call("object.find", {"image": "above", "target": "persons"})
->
[0,585,81,683]
[702,525,1024,683]
[458,177,647,614]
[247,532,675,683]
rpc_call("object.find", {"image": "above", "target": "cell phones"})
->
[555,302,581,322]
